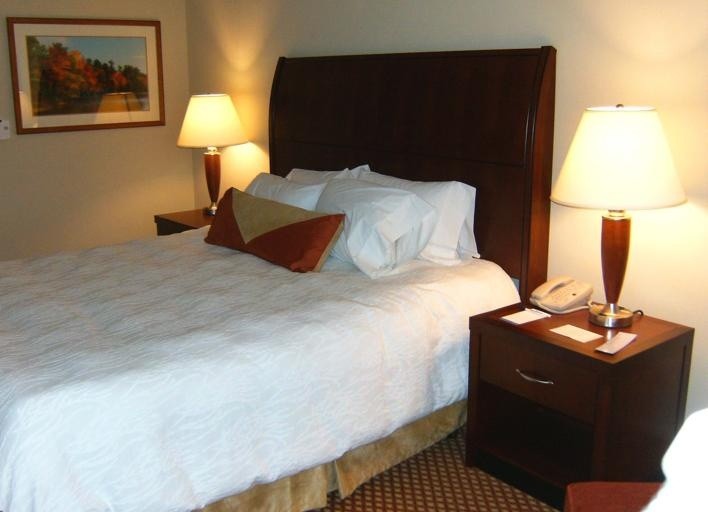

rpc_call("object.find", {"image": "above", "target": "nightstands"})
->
[464,298,695,512]
[154,209,214,236]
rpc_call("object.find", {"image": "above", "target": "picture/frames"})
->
[6,17,165,135]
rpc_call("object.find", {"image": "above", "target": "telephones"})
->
[529,276,593,313]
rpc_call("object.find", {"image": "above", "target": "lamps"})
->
[176,93,246,216]
[550,103,687,329]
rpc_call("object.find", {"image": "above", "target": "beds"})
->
[0,46,557,512]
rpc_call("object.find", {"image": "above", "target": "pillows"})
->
[204,187,345,273]
[243,172,329,212]
[356,167,480,267]
[285,165,371,185]
[316,178,438,280]
